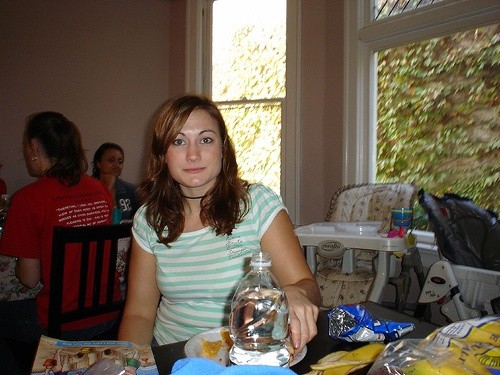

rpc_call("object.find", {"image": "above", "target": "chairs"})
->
[294,182,431,322]
[47,224,133,340]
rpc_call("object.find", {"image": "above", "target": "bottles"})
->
[229,251,290,369]
[0,195,11,232]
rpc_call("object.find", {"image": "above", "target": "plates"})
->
[184,326,307,367]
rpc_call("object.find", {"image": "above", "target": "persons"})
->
[0,111,120,340]
[93,143,144,224]
[117,95,321,354]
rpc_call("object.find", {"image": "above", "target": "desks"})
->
[151,301,445,375]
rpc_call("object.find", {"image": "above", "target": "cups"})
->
[113,209,123,225]
[391,207,413,226]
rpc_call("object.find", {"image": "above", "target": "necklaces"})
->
[179,192,215,199]
